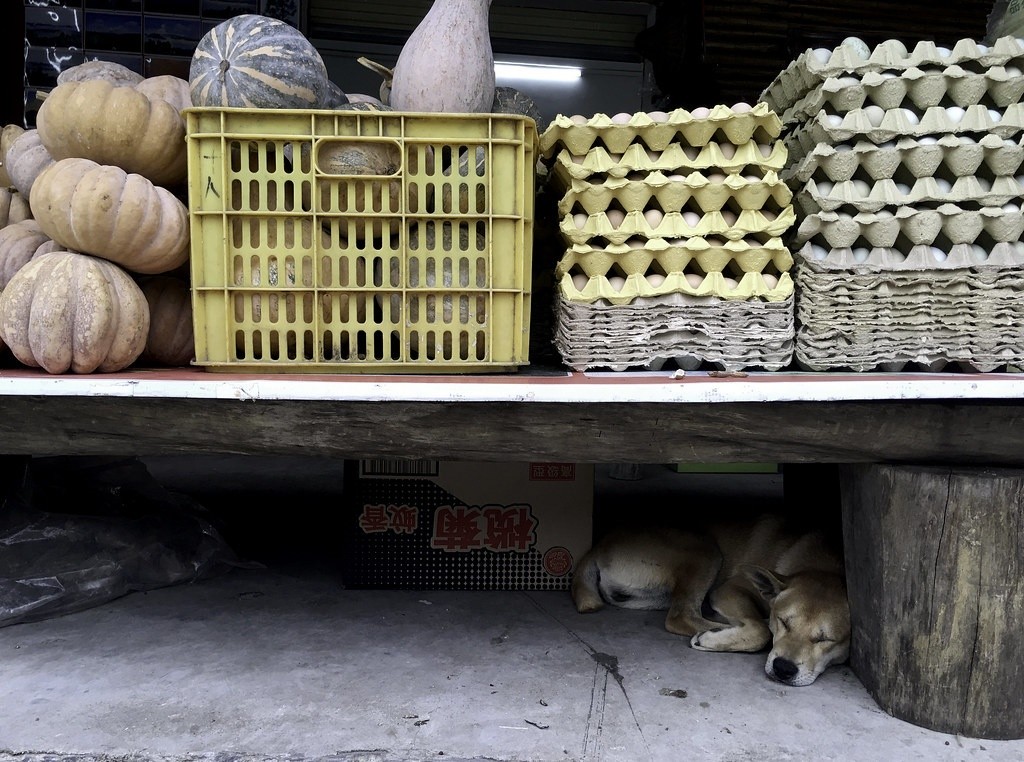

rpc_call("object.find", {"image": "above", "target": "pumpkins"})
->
[0,0,546,377]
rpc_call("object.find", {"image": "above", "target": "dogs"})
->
[569,496,852,686]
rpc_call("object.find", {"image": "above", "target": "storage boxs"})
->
[343,459,594,591]
[181,106,540,375]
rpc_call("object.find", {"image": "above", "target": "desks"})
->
[0,367,1024,741]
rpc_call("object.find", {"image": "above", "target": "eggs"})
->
[571,100,779,290]
[805,36,1023,261]
